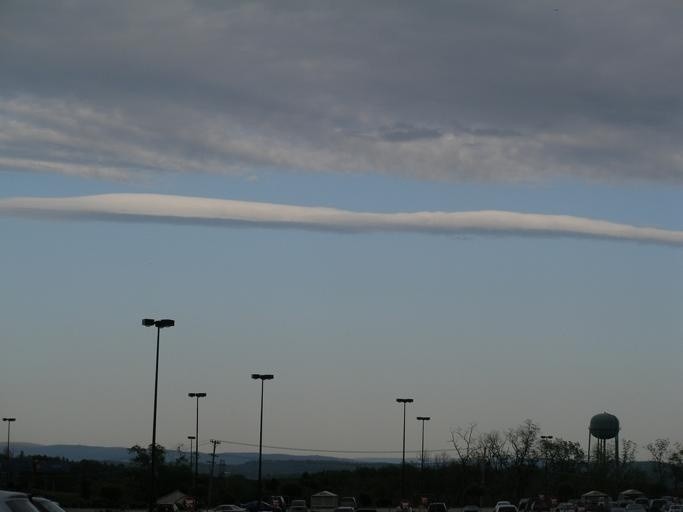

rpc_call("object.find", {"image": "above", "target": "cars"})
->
[334,496,357,512]
[427,499,682,512]
[213,496,306,512]
[0,490,66,512]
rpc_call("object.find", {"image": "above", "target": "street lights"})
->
[187,391,205,495]
[394,399,432,502]
[249,374,274,508]
[539,435,553,499]
[139,318,176,512]
[1,418,16,489]
